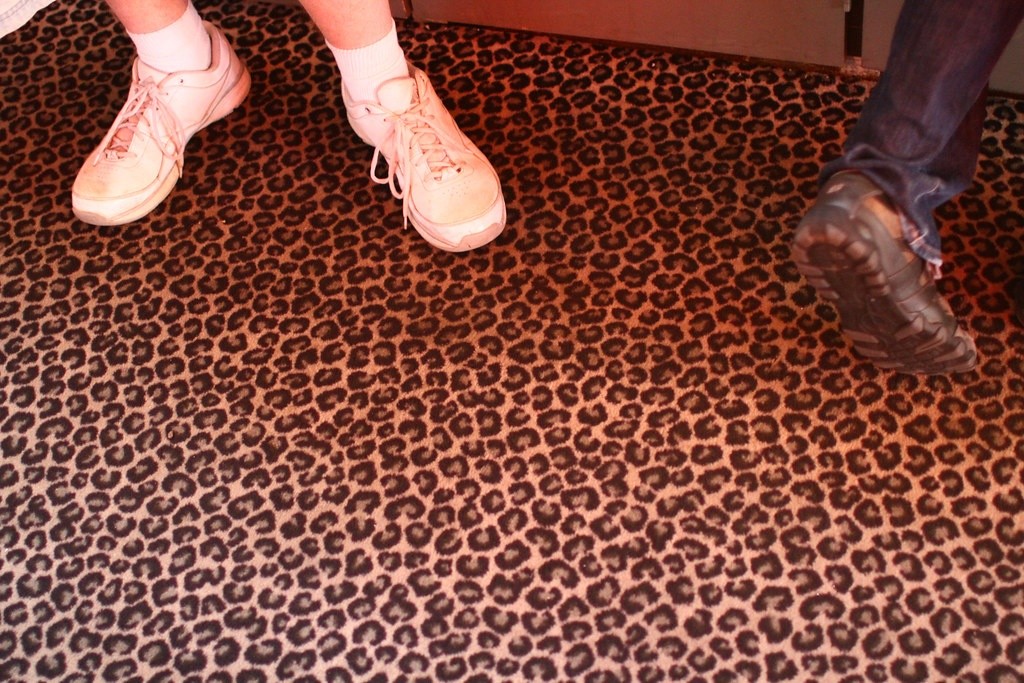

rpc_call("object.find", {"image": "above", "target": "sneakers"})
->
[71,18,252,226]
[340,61,506,252]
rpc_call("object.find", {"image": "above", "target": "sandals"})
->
[791,166,977,372]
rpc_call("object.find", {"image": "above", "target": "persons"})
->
[794,0,1024,378]
[70,0,506,254]
